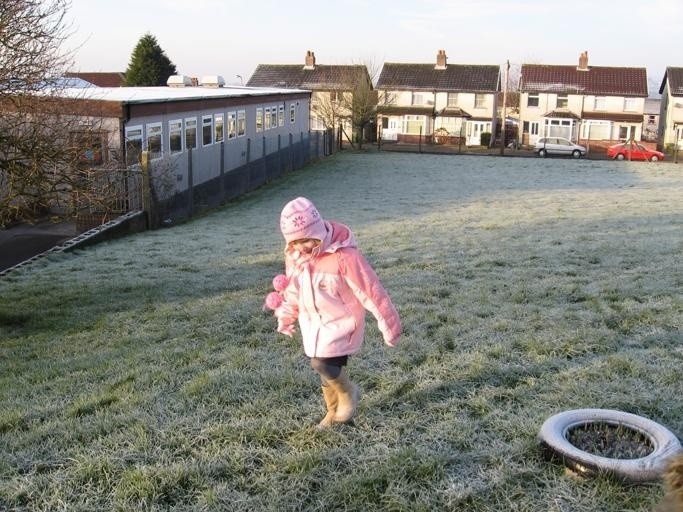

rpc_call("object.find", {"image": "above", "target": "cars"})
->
[534,137,667,162]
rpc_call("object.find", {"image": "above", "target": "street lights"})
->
[235,75,242,84]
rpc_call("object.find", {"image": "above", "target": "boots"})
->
[317,381,339,429]
[329,368,360,423]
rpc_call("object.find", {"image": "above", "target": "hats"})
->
[279,196,328,245]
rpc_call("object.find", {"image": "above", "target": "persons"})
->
[265,196,401,433]
